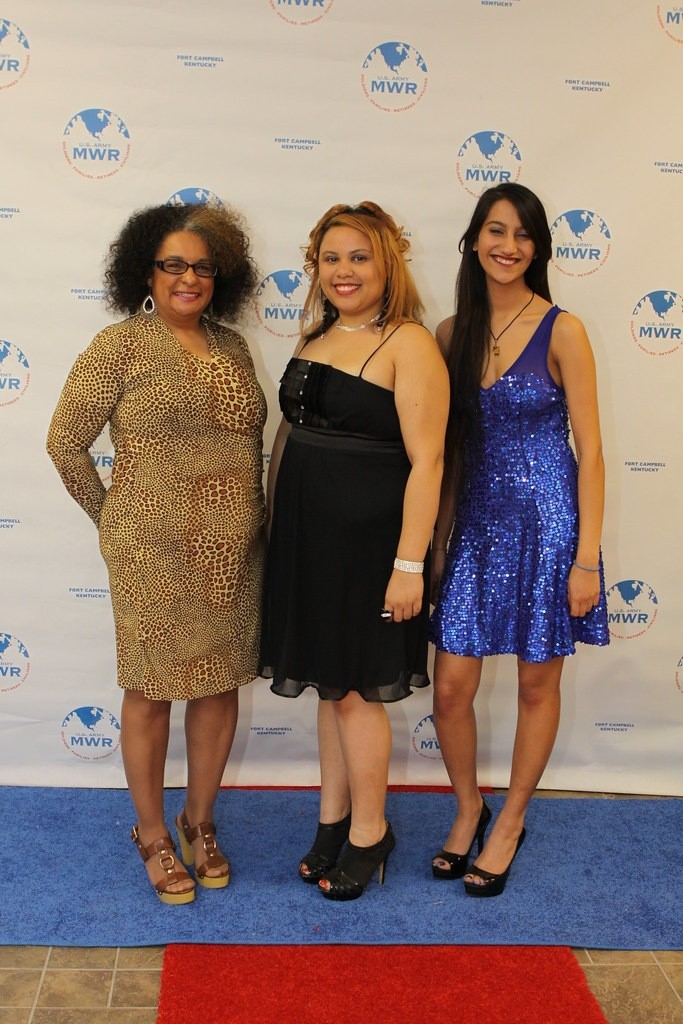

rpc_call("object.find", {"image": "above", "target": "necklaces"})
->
[486,291,535,357]
[336,309,385,332]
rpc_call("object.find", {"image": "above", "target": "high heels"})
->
[432,796,493,879]
[298,812,352,883]
[174,809,229,888]
[318,820,396,901]
[129,825,194,904]
[464,826,526,897]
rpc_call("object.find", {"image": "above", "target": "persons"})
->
[432,182,611,897]
[257,201,451,901]
[45,201,268,905]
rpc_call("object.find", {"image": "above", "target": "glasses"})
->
[152,258,217,278]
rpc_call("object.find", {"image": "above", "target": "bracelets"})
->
[573,561,600,571]
[393,558,424,573]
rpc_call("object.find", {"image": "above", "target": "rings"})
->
[381,608,393,619]
